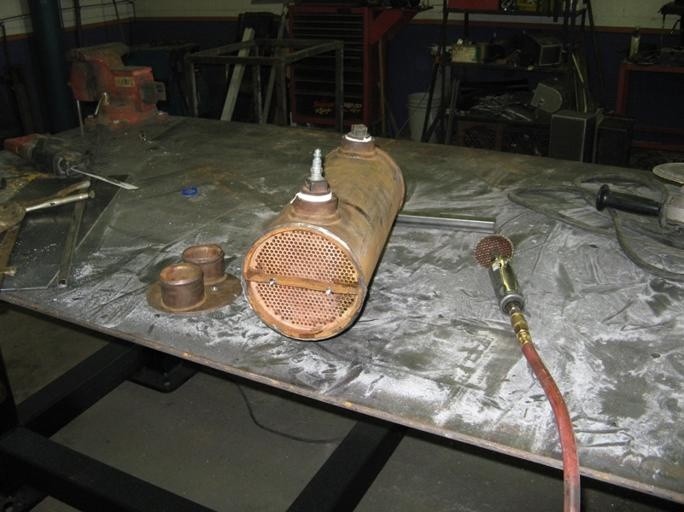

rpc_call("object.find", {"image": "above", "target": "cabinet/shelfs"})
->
[420,0,591,144]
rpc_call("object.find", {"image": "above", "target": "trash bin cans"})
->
[406,92,443,144]
